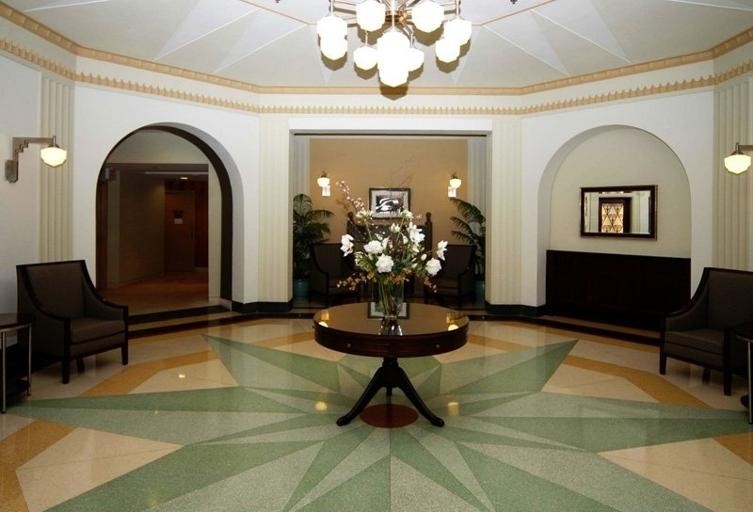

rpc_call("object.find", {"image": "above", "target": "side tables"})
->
[734,329,753,424]
[1,313,34,413]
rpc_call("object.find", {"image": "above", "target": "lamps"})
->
[317,0,472,88]
[3,136,67,183]
[173,210,184,225]
[317,171,331,197]
[448,172,461,198]
[724,142,753,174]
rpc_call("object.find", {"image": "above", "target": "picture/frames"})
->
[581,184,658,239]
[368,187,411,219]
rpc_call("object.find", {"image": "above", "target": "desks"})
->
[312,301,469,427]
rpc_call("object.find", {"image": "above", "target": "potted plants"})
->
[292,194,334,301]
[449,198,485,309]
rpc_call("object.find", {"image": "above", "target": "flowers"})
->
[335,180,450,318]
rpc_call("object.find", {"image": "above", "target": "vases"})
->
[380,316,403,337]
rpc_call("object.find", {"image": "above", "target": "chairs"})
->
[17,260,130,384]
[423,244,477,310]
[659,268,753,395]
[310,243,361,308]
